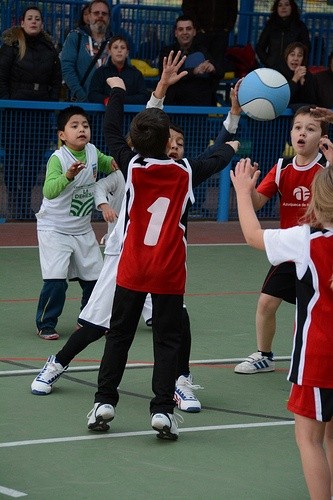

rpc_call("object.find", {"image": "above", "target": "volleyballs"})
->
[239,68,291,121]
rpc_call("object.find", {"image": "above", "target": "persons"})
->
[88,34,148,217]
[182,0,238,60]
[35,105,120,339]
[158,14,227,216]
[255,0,311,67]
[230,158,333,500]
[87,76,241,439]
[0,7,62,218]
[257,41,333,216]
[30,50,245,412]
[61,0,117,103]
[234,104,333,374]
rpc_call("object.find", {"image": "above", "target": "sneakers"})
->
[37,326,59,340]
[31,355,70,395]
[173,375,203,413]
[150,411,181,440]
[234,351,276,374]
[87,401,115,432]
